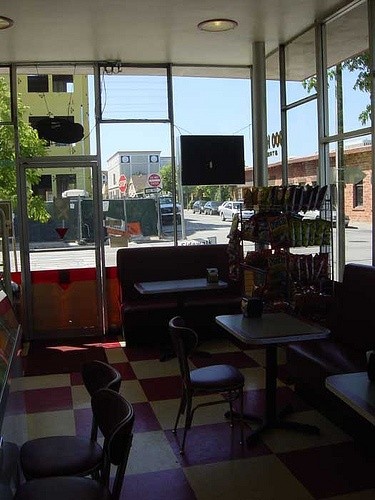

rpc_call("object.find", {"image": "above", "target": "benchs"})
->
[116,241,245,349]
[290,266,375,457]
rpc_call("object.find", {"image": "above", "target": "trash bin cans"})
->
[108,222,129,247]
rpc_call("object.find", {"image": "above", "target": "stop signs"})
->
[148,174,161,186]
[119,175,127,193]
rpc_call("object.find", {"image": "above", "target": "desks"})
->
[134,277,228,360]
[325,371,375,427]
[215,310,331,447]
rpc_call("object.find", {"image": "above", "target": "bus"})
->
[62,190,89,208]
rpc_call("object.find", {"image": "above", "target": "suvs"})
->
[156,196,180,225]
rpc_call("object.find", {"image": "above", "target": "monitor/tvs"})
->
[179,135,245,185]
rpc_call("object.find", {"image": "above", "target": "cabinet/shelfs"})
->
[235,189,339,313]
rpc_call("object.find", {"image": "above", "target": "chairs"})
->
[166,313,249,456]
[15,388,137,500]
[20,359,122,480]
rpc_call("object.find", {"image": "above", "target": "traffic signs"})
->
[144,187,163,195]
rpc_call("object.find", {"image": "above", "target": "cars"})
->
[188,199,254,222]
[298,206,350,227]
